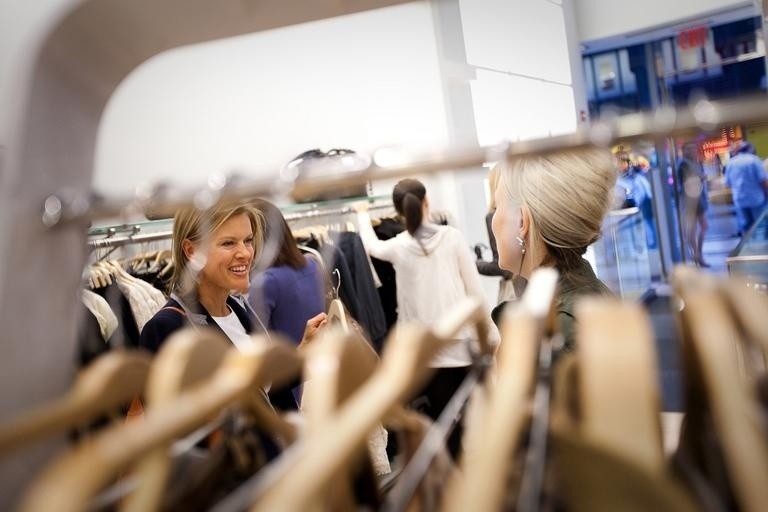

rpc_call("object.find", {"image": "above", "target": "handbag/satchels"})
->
[112,308,226,512]
[283,148,367,203]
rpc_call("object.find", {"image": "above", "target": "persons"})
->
[243,197,326,350]
[488,134,617,359]
[354,176,490,407]
[610,150,658,250]
[137,191,278,465]
[722,140,768,242]
[675,138,712,269]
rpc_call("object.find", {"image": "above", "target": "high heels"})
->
[694,256,711,267]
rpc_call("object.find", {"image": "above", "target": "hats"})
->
[732,140,750,153]
